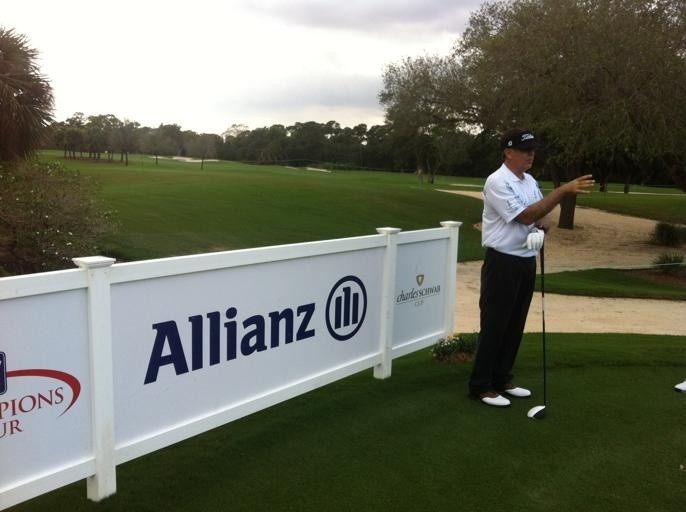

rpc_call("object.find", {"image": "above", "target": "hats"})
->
[501,128,543,151]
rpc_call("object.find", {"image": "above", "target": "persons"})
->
[469,128,597,408]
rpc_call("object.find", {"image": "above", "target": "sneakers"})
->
[504,386,532,397]
[476,390,511,407]
[674,379,686,393]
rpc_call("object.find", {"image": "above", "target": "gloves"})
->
[521,227,545,251]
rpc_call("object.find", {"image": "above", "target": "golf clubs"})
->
[527,244,546,419]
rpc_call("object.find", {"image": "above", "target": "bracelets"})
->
[535,223,549,232]
